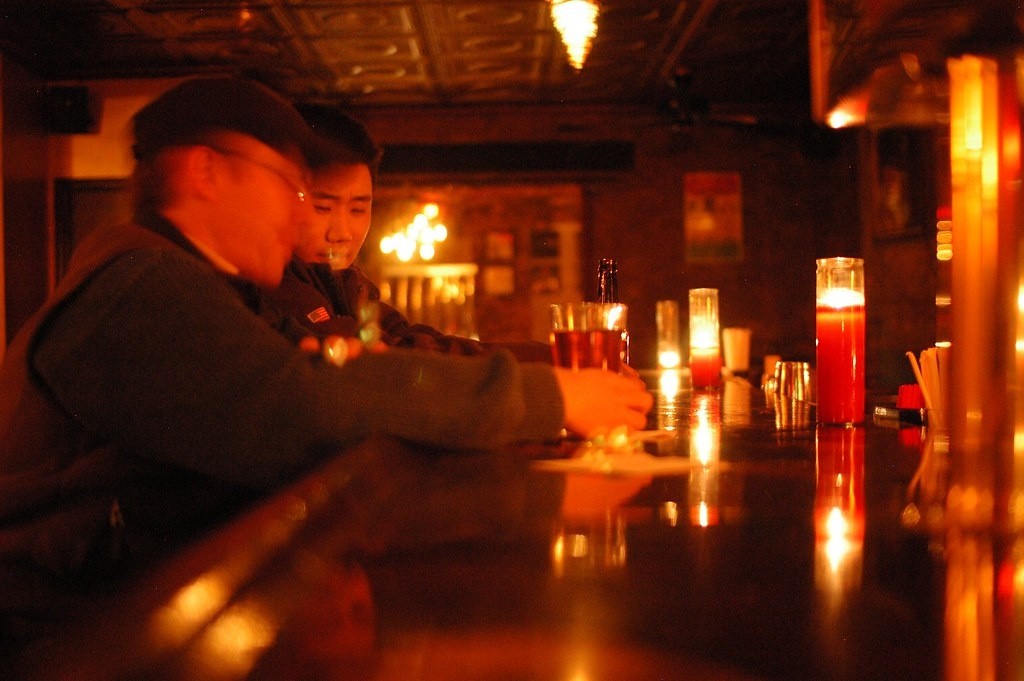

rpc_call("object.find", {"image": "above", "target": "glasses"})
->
[204,142,310,211]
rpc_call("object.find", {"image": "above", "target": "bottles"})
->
[597,258,618,304]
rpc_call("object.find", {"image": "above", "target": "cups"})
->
[776,362,812,401]
[816,258,865,426]
[723,329,750,372]
[688,288,722,388]
[551,304,629,437]
[656,301,680,368]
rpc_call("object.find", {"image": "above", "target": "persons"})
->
[1,75,653,681]
[246,100,645,393]
